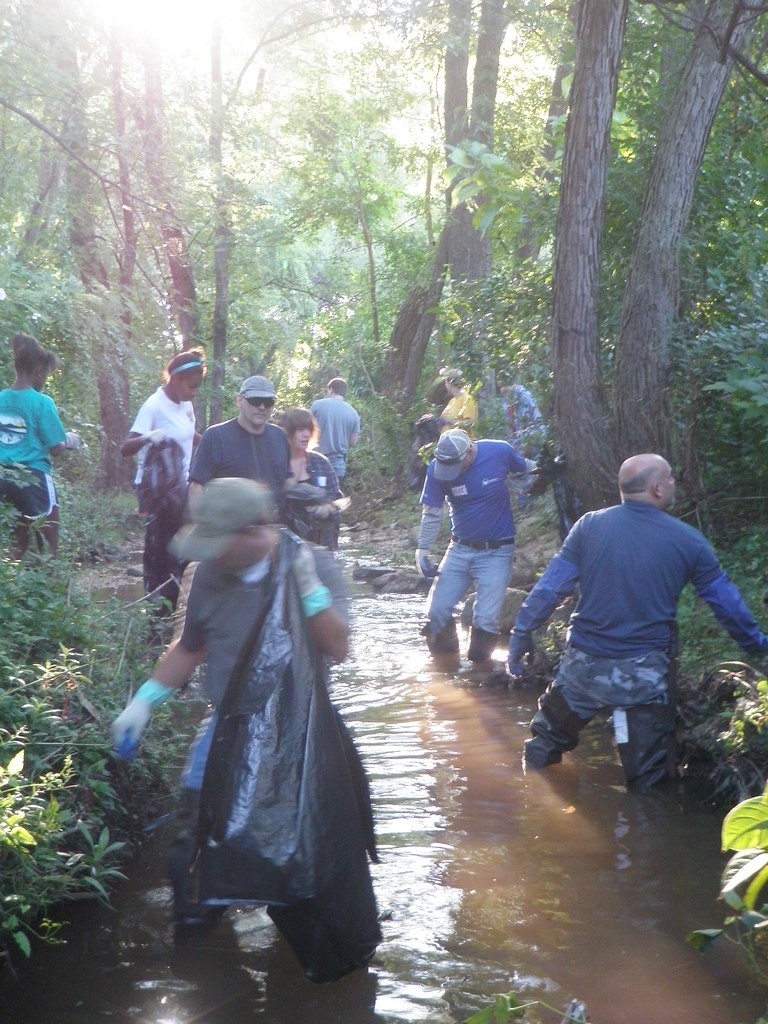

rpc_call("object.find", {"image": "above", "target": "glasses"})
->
[242,396,275,409]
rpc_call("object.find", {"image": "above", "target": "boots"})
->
[425,618,460,670]
[468,627,499,666]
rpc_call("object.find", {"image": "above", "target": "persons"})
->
[415,429,567,662]
[120,346,207,646]
[187,375,295,525]
[0,333,80,560]
[278,408,352,552]
[309,378,360,489]
[507,453,768,800]
[437,368,478,441]
[109,477,383,984]
[498,375,548,524]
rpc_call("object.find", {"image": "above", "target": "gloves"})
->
[109,678,177,759]
[507,558,578,679]
[292,546,333,618]
[66,430,81,450]
[697,574,768,667]
[415,547,439,579]
[141,428,169,447]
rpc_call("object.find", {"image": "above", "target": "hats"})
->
[167,476,271,563]
[432,428,470,482]
[241,375,278,401]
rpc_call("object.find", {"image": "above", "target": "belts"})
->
[450,535,515,550]
